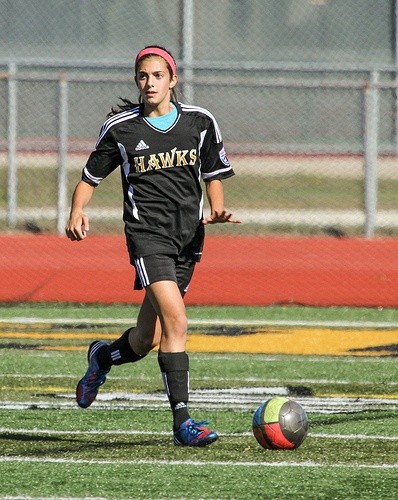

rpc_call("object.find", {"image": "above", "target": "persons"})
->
[65,45,240,446]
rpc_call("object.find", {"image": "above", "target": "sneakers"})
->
[173,418,218,447]
[75,340,111,409]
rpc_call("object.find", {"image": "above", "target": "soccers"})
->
[251,396,308,448]
